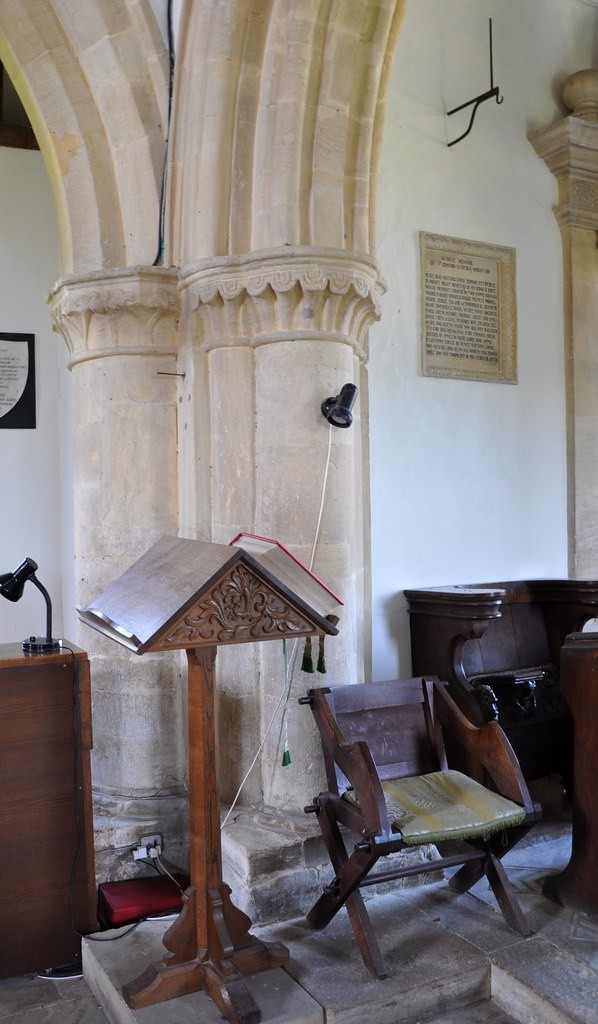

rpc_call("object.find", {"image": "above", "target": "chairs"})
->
[297,674,536,980]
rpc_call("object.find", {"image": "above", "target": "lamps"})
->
[0,557,61,653]
[319,382,358,429]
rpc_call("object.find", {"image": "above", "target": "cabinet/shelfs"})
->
[0,638,97,979]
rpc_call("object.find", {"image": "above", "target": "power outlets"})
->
[139,832,163,857]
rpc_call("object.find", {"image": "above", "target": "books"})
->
[228,531,345,620]
